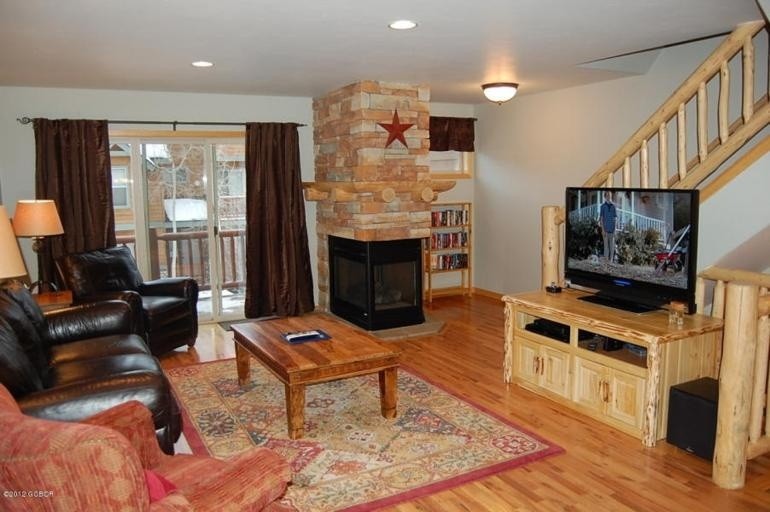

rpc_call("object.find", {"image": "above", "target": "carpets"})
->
[162,357,566,511]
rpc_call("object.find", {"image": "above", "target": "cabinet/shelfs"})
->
[422,200,474,300]
[500,284,724,447]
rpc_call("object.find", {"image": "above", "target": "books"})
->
[431,231,468,249]
[432,210,469,227]
[430,253,468,271]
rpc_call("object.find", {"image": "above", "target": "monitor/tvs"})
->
[563,186,700,314]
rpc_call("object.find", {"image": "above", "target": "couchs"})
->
[0,384,290,512]
[0,277,182,458]
[49,246,198,358]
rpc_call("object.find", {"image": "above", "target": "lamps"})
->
[0,205,27,290]
[481,82,519,105]
[13,200,65,292]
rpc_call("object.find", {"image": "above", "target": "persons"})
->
[600,190,618,262]
